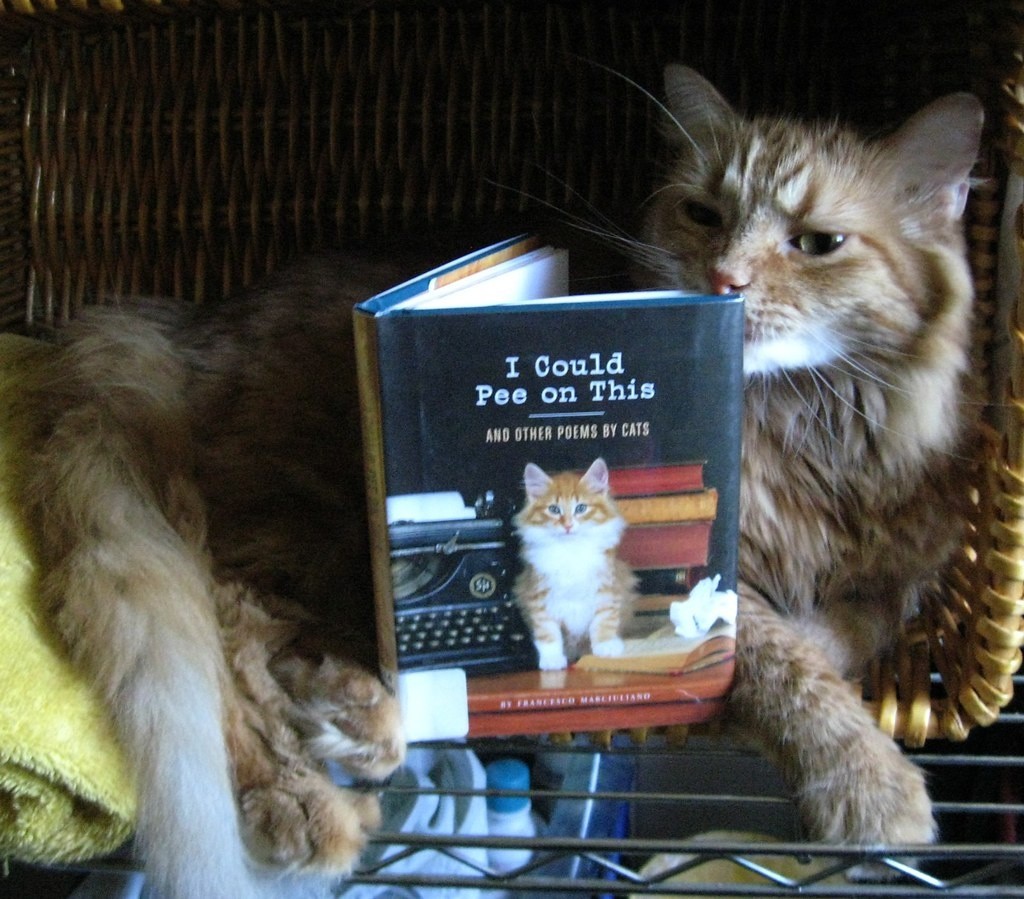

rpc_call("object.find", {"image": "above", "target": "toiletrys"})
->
[484,758,548,878]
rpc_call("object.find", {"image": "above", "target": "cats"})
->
[13,61,988,899]
[510,456,632,668]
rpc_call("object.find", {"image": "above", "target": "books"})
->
[352,230,746,744]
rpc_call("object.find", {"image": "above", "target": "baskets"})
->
[0,1,1024,767]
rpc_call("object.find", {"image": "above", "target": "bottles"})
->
[486,760,536,876]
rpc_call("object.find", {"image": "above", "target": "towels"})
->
[0,327,142,873]
